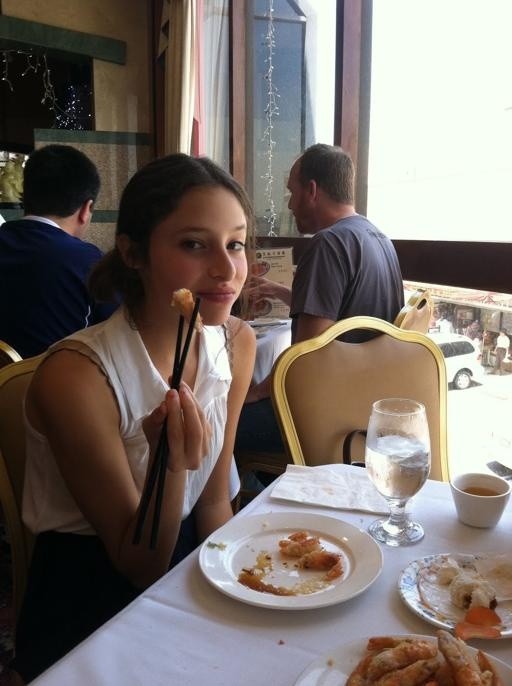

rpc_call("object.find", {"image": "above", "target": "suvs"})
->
[426,332,483,395]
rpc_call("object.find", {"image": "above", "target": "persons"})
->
[429,308,510,377]
[0,143,127,363]
[10,152,257,682]
[229,143,403,519]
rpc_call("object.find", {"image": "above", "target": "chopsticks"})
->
[132,295,202,550]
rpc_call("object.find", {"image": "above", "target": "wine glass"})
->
[364,398,433,548]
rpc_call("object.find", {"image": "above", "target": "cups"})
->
[449,473,511,529]
[246,281,260,296]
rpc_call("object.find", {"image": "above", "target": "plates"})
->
[198,512,385,614]
[291,634,512,686]
[250,299,273,315]
[249,258,270,278]
[398,552,512,640]
[249,315,292,339]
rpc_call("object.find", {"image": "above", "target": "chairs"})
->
[388,283,436,344]
[1,340,104,629]
[270,312,460,490]
